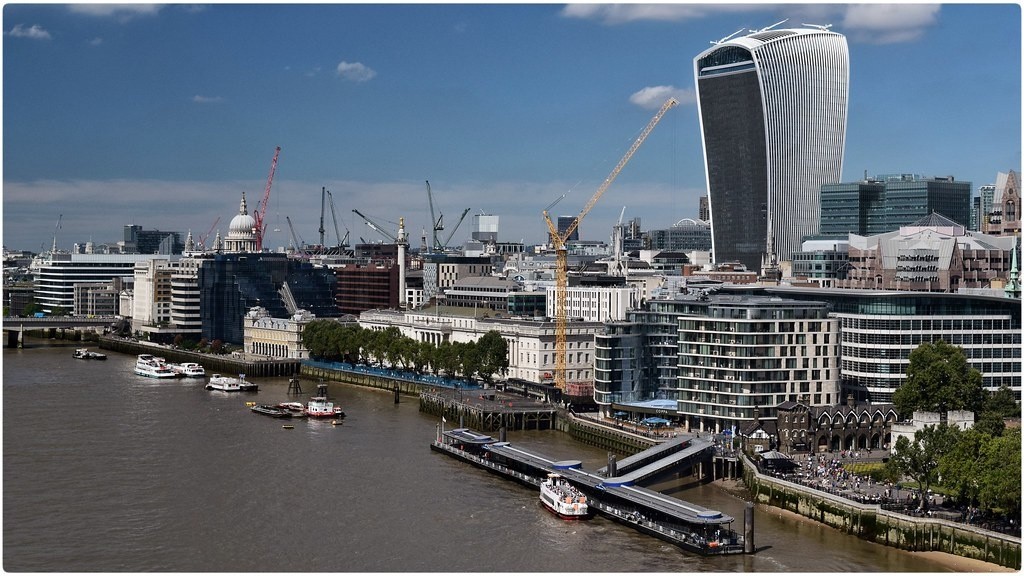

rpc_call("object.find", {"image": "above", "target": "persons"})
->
[708,426,713,436]
[479,452,489,461]
[697,431,700,438]
[774,448,868,490]
[628,417,684,429]
[961,505,1021,533]
[704,529,720,540]
[854,489,937,517]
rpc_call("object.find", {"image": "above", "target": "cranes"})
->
[542,96,681,389]
[287,181,471,254]
[196,215,222,250]
[53,213,63,257]
[254,147,282,251]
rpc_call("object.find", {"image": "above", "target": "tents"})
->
[615,411,628,415]
[640,417,672,423]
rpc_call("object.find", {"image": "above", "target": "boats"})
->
[167,363,206,377]
[204,374,241,392]
[229,374,259,393]
[540,473,590,521]
[72,348,110,361]
[135,354,180,379]
[253,395,348,420]
